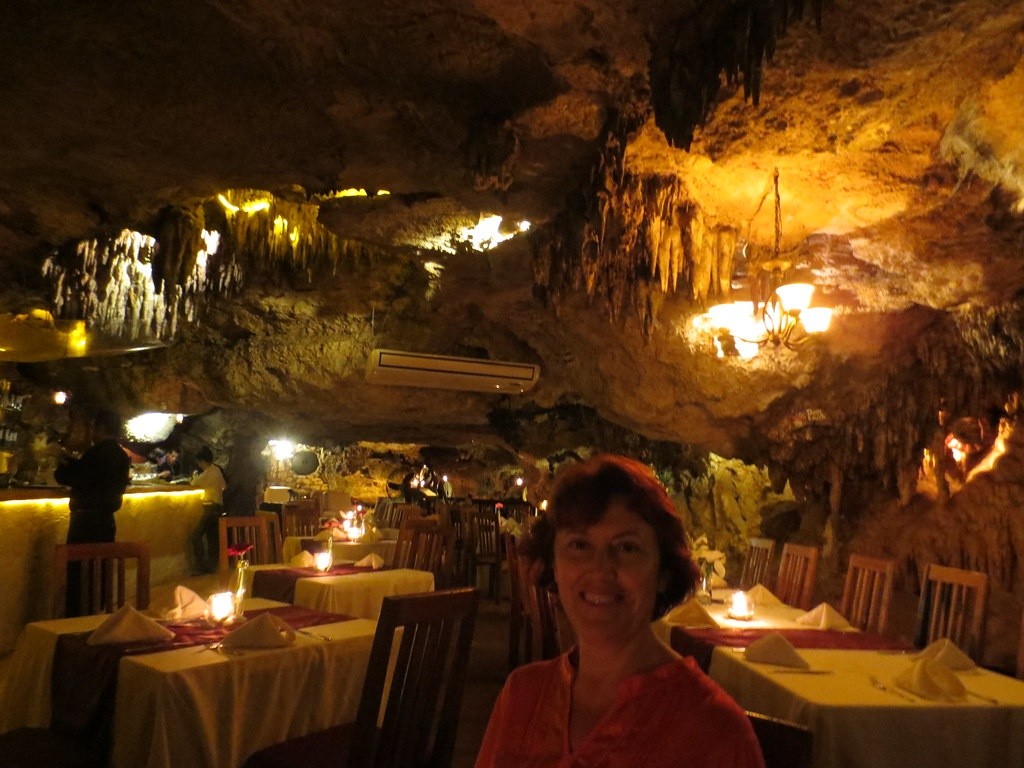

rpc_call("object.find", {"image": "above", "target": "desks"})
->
[1,532,433,768]
[654,589,1024,768]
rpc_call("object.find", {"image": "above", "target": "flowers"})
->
[495,503,503,510]
[226,543,256,605]
[325,520,339,534]
[689,533,727,580]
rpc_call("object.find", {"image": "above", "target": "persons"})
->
[479,454,768,768]
[154,448,182,481]
[51,411,132,618]
[170,447,229,577]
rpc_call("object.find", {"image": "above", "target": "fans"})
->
[386,467,421,505]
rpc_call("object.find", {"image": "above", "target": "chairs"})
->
[48,542,156,617]
[219,493,572,768]
[740,537,989,663]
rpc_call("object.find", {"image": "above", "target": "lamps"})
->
[708,166,835,349]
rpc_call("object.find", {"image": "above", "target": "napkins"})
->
[149,584,209,620]
[220,613,296,646]
[86,604,177,644]
[291,550,315,568]
[672,600,975,700]
[355,553,384,569]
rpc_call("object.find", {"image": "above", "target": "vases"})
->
[697,561,714,606]
[228,559,255,616]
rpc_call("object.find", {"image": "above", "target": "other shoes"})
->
[191,568,207,576]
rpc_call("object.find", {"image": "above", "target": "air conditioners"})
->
[365,304,540,393]
